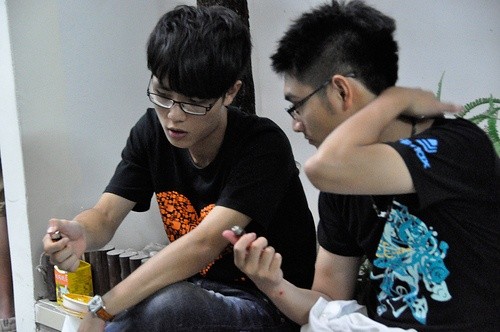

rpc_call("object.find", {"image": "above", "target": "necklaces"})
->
[406,115,420,141]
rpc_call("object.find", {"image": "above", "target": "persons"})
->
[27,4,319,332]
[221,0,500,332]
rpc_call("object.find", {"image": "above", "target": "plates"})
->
[61,293,94,312]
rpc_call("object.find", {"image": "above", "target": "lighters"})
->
[231,225,264,254]
[49,229,69,260]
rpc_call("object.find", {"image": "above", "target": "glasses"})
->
[284,73,357,120]
[147,72,220,116]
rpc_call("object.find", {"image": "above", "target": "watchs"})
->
[86,293,117,324]
